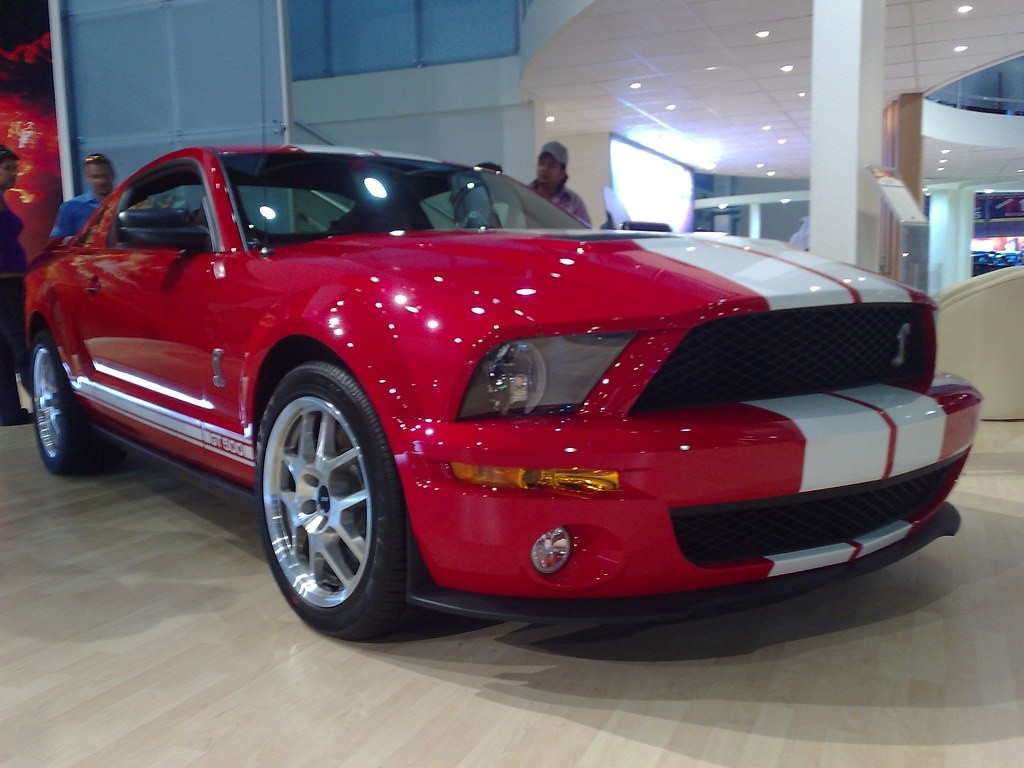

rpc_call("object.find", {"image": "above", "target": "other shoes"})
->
[0,408,34,427]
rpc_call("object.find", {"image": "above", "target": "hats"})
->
[537,141,568,165]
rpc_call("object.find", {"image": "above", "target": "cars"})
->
[11,142,980,646]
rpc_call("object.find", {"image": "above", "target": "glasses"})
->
[85,155,107,163]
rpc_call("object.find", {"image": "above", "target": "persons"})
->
[327,174,434,234]
[454,162,503,229]
[0,142,35,426]
[508,141,592,230]
[47,153,115,241]
[129,195,188,210]
